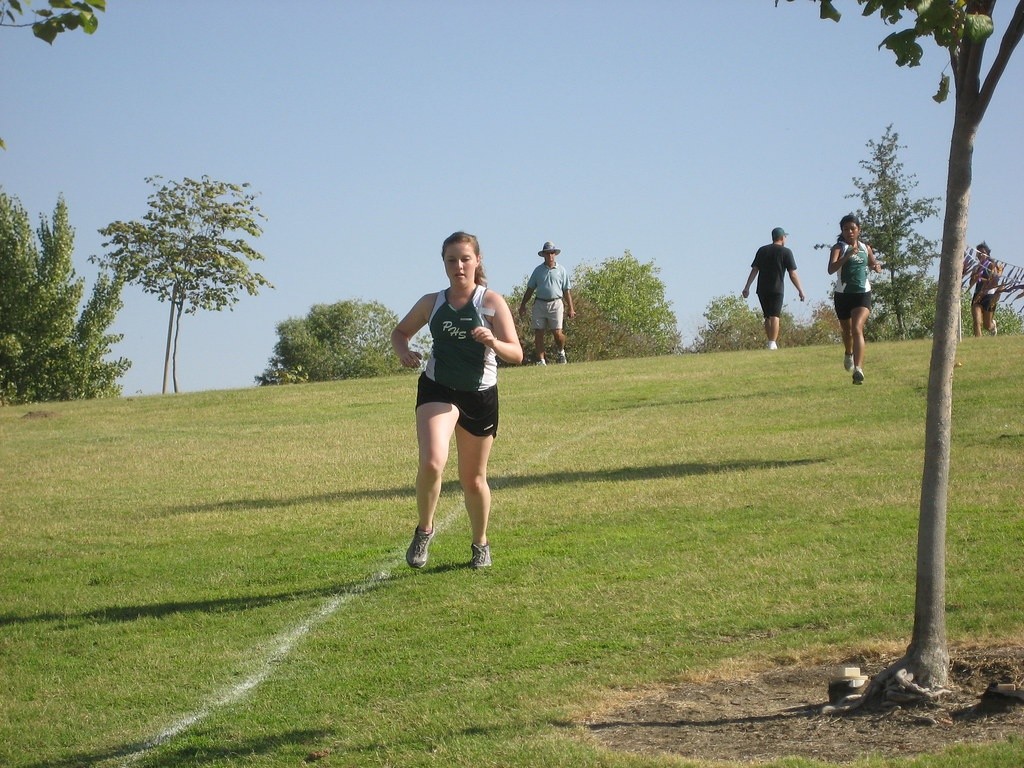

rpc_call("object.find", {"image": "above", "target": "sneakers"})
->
[852,368,864,385]
[471,541,492,570]
[844,351,854,371]
[406,520,435,569]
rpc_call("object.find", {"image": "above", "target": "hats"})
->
[772,227,789,241]
[538,241,561,257]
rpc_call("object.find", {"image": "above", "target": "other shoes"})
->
[556,352,566,365]
[536,360,546,367]
[990,319,997,336]
[768,341,777,350]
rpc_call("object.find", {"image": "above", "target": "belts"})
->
[535,297,562,302]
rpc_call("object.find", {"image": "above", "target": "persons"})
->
[969,243,1002,337]
[827,216,882,385]
[742,227,805,350]
[519,241,575,365]
[392,231,523,569]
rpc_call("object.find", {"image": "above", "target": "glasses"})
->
[784,235,786,238]
[543,251,555,255]
